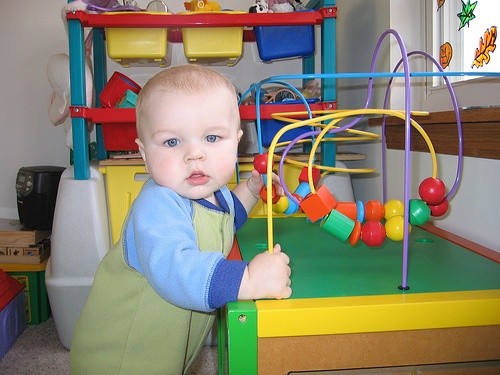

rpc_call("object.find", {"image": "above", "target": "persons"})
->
[69,65,293,375]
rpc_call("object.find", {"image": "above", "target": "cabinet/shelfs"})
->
[45,0,366,353]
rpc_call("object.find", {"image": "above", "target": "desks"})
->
[214,213,500,375]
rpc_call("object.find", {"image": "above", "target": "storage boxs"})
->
[0,256,50,325]
[255,97,320,149]
[101,123,139,152]
[178,11,249,67]
[102,12,175,68]
[253,8,315,64]
